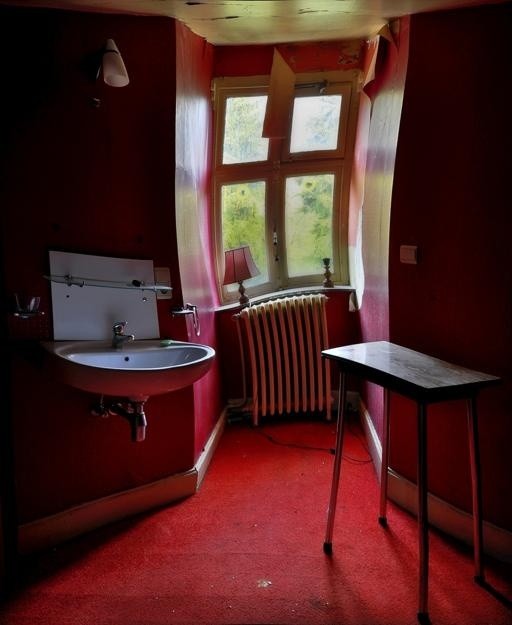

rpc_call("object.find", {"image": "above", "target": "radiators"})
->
[231,293,336,425]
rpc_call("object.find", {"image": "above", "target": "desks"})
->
[314,341,502,625]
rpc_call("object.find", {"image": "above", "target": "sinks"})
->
[51,339,217,403]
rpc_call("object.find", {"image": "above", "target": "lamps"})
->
[221,245,261,305]
[87,34,132,110]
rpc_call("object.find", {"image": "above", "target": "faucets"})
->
[113,320,135,350]
